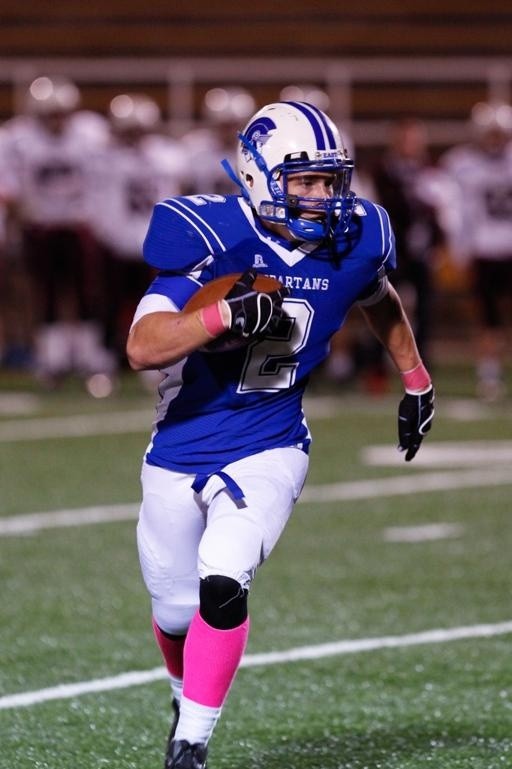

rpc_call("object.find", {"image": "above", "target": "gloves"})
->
[222,270,289,337]
[397,383,435,462]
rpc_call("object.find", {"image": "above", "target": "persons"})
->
[1,79,511,393]
[126,102,434,769]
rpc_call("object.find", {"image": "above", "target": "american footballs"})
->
[180,274,284,353]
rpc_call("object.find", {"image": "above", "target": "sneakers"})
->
[164,696,208,769]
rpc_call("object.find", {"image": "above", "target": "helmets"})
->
[235,99,358,245]
